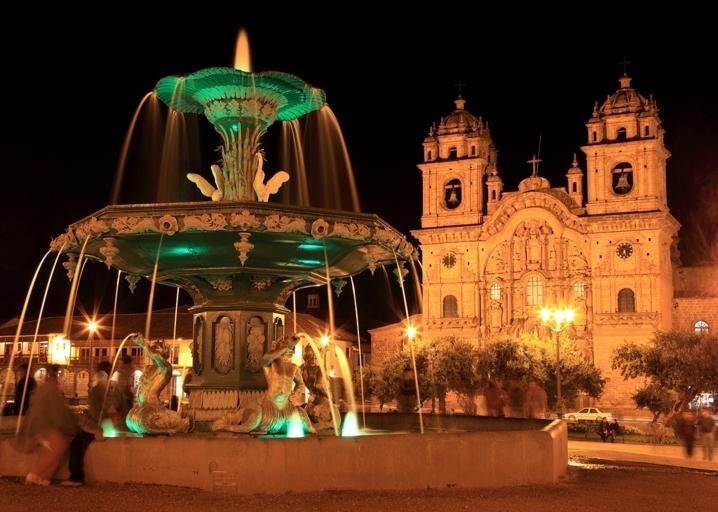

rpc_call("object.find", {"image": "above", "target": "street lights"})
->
[87,322,97,395]
[540,310,573,418]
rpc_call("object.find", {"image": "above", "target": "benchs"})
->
[584,424,625,442]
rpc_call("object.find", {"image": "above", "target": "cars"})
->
[563,407,612,421]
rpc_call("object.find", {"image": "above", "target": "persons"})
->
[124,332,189,436]
[292,342,347,432]
[1,362,37,416]
[60,360,127,486]
[19,362,79,486]
[212,335,314,433]
[609,419,619,443]
[598,416,610,441]
